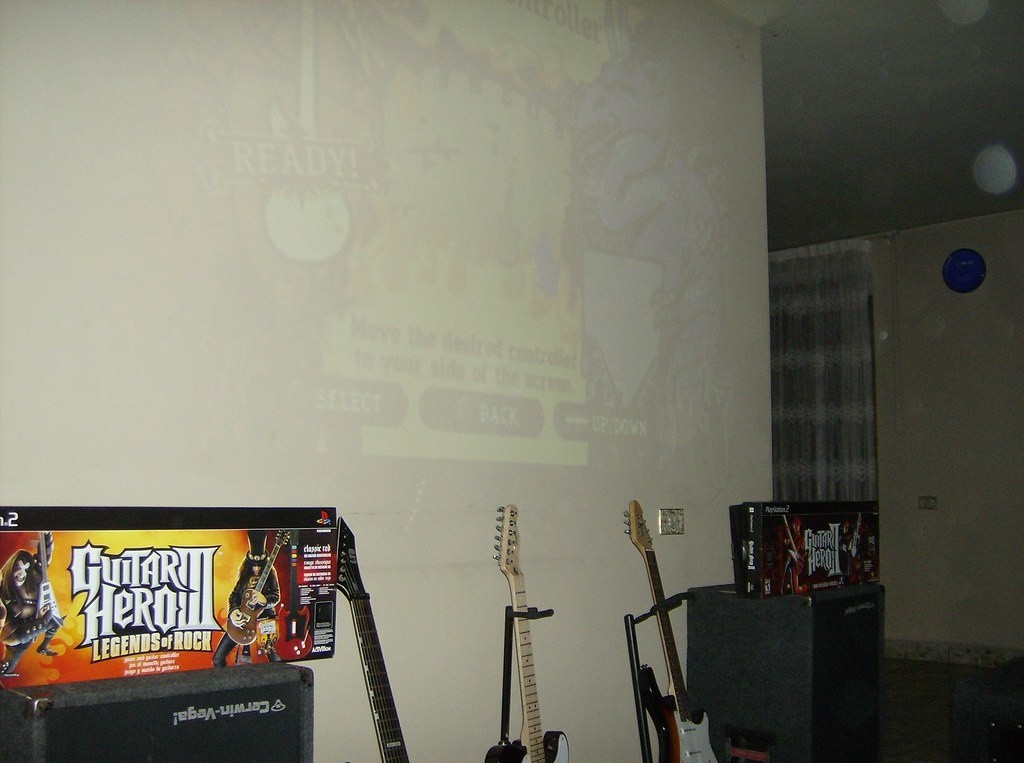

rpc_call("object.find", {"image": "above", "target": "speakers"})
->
[686,585,886,763]
[0,661,315,763]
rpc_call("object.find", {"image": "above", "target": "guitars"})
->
[35,532,64,626]
[783,514,808,575]
[276,530,313,660]
[492,504,570,763]
[225,530,292,645]
[335,516,412,763]
[848,513,862,557]
[623,500,719,763]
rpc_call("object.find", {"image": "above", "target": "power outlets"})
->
[918,496,937,510]
[659,508,684,535]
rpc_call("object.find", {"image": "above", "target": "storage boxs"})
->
[728,501,880,601]
[0,506,337,690]
